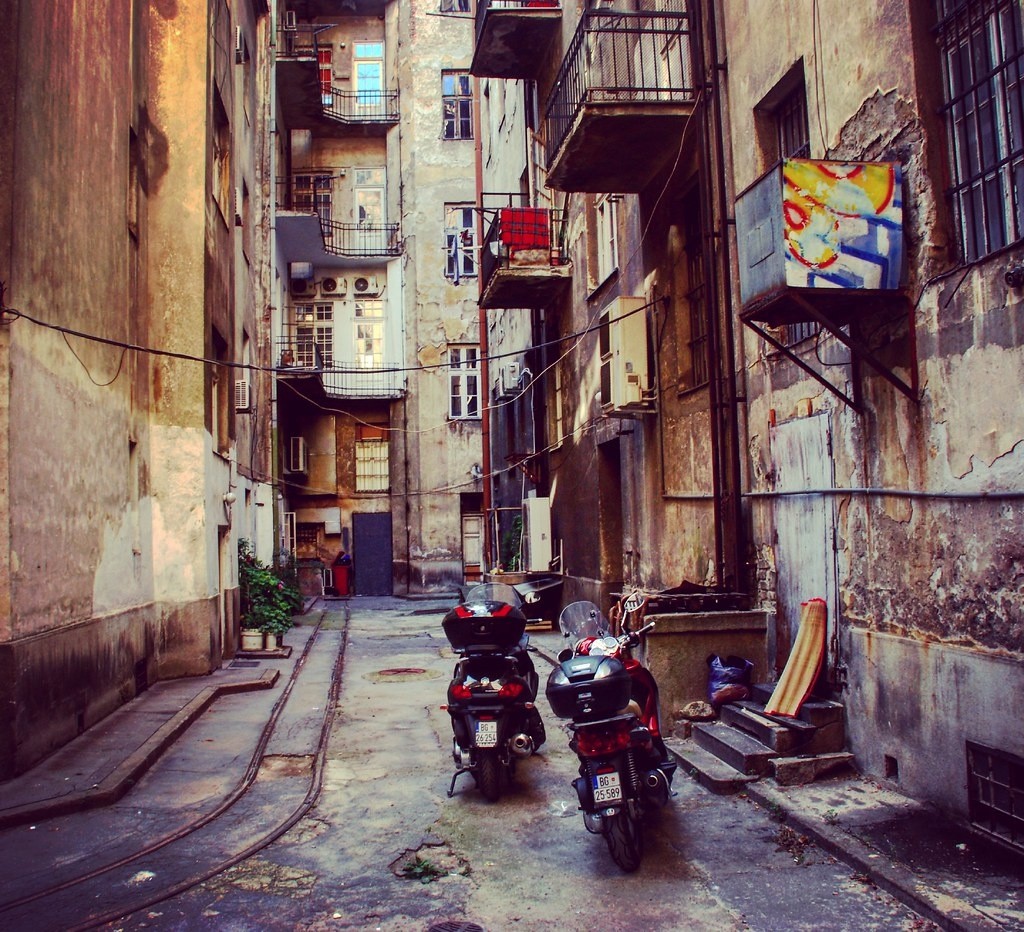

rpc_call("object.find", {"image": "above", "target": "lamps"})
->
[290,436,308,476]
[223,492,236,505]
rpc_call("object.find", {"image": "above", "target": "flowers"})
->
[236,538,298,632]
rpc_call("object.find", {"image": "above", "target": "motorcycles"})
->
[445,580,547,800]
[526,589,676,872]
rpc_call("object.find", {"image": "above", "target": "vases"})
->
[240,631,264,650]
[266,632,276,649]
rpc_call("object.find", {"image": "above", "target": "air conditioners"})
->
[494,376,513,401]
[353,275,379,294]
[235,379,253,414]
[235,25,248,65]
[502,362,523,393]
[320,277,347,294]
